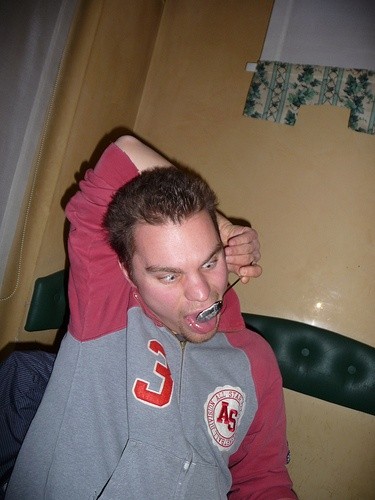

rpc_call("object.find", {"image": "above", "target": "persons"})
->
[3,134,300,500]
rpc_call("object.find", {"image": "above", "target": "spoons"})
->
[196,276,251,323]
